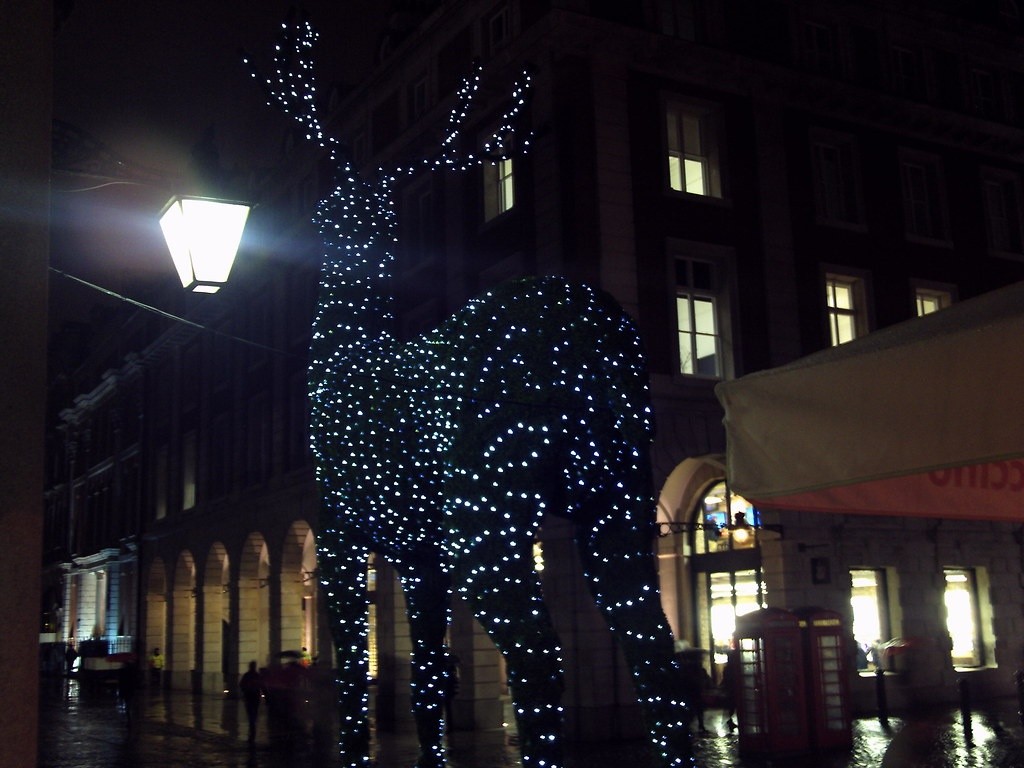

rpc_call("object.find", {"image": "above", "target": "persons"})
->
[689,652,713,730]
[66,645,79,669]
[240,657,319,734]
[719,650,744,729]
[854,641,868,669]
[442,645,460,733]
[147,648,165,695]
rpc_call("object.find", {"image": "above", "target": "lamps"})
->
[54,118,255,293]
[727,513,781,542]
[656,520,721,542]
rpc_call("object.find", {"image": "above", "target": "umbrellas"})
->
[274,651,304,658]
[716,280,1024,522]
[677,648,706,653]
